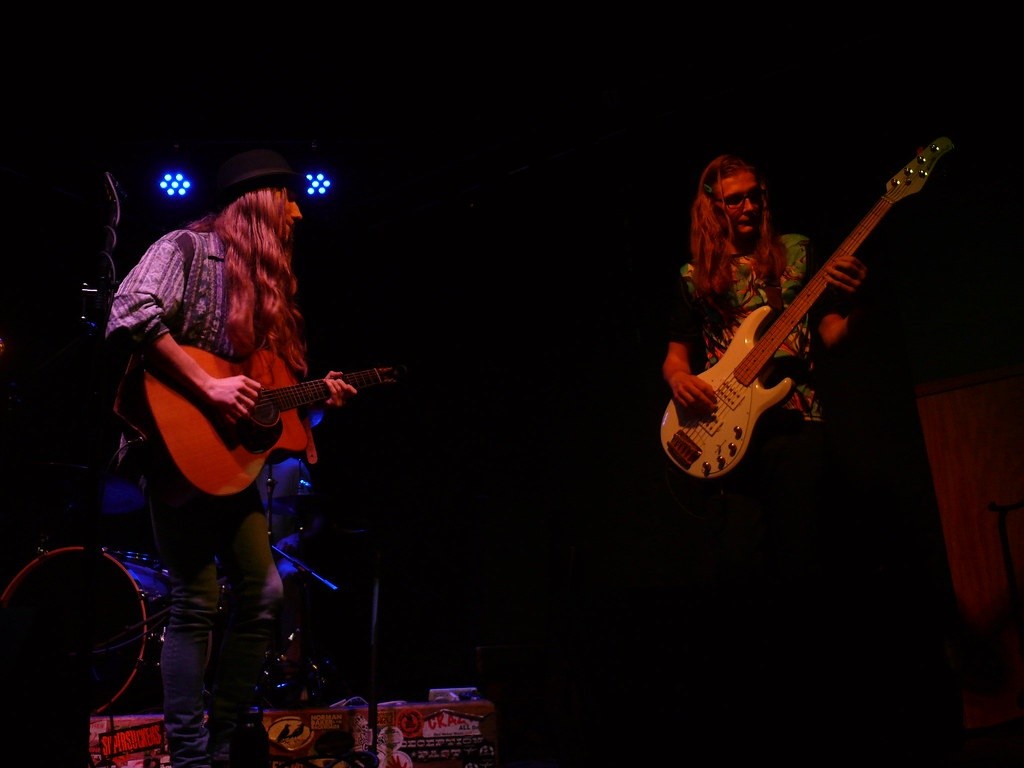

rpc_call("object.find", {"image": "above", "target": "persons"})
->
[106,143,357,768]
[663,156,868,751]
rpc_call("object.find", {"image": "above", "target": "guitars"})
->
[658,138,955,480]
[121,341,405,499]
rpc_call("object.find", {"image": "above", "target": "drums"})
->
[0,541,175,716]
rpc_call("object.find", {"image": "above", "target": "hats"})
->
[213,149,305,196]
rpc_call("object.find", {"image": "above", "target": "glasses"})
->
[712,185,763,209]
